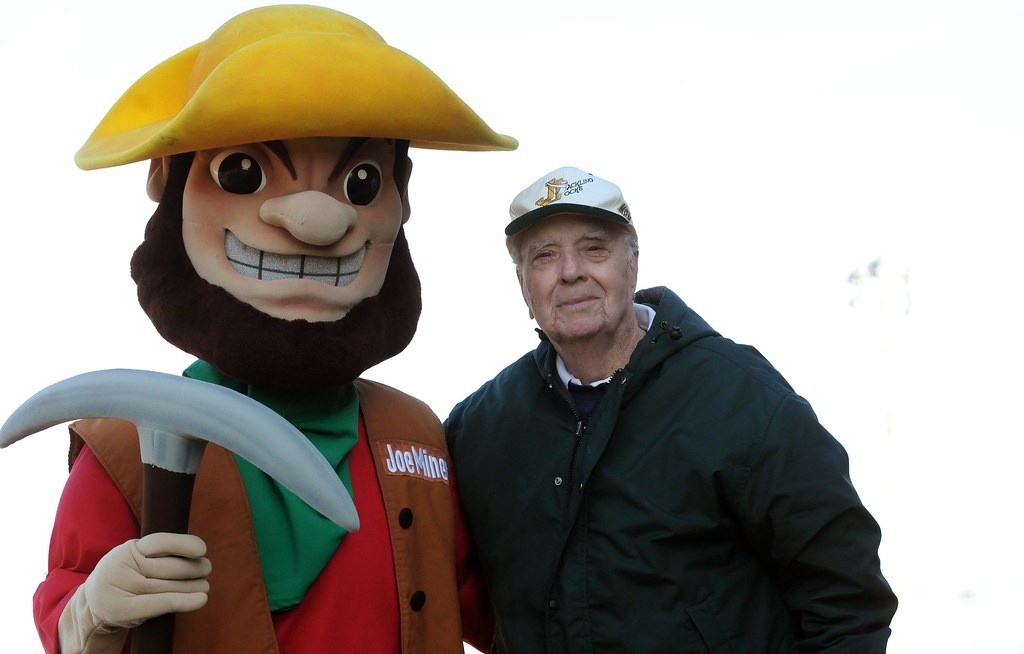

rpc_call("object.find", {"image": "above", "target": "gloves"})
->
[55,530,213,653]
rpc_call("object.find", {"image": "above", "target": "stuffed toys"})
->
[3,7,517,653]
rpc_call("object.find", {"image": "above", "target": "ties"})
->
[566,380,610,443]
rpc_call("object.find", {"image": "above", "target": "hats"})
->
[71,5,517,170]
[503,164,636,238]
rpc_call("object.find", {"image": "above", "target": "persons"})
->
[444,168,899,654]
